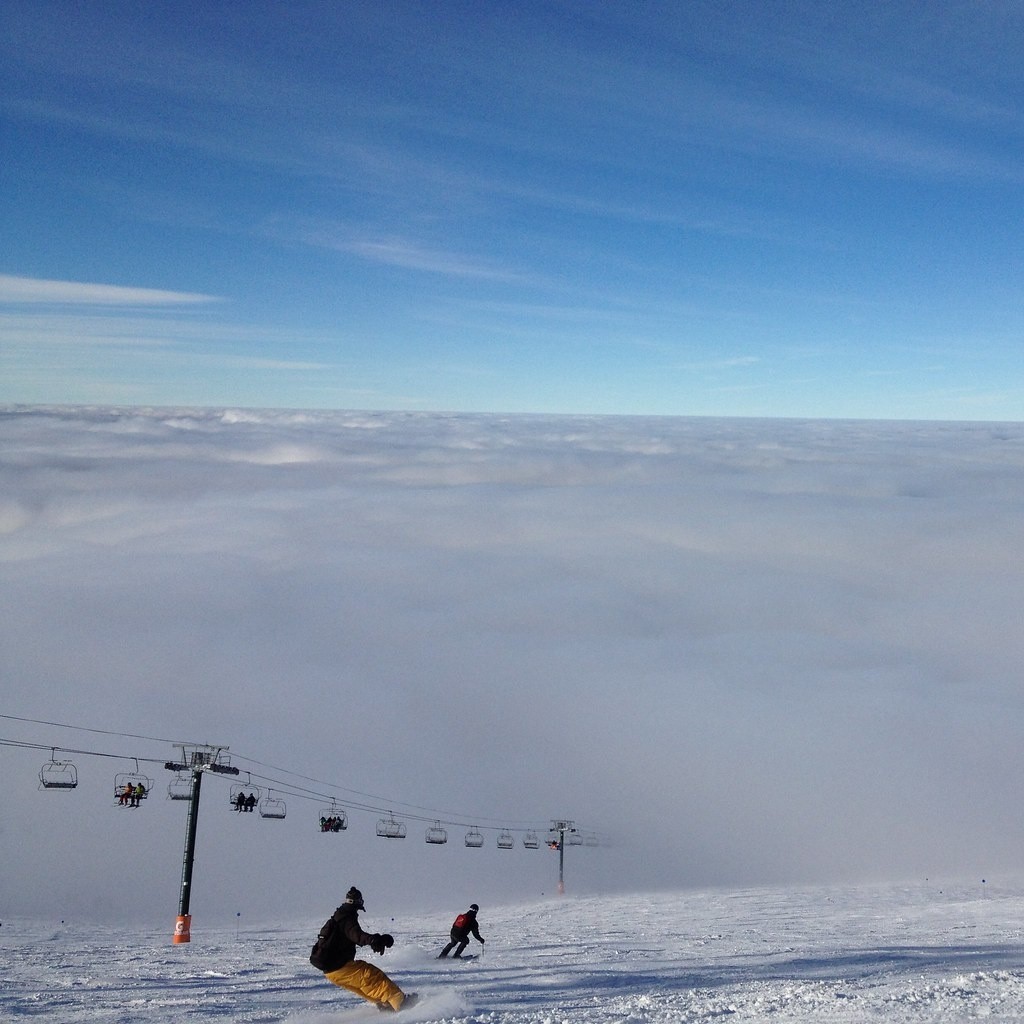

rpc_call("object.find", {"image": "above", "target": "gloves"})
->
[480,938,484,943]
[370,933,394,956]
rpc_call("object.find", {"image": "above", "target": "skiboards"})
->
[430,955,481,966]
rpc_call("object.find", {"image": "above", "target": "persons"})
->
[119,781,146,808]
[311,885,418,1011]
[235,792,256,814]
[439,904,485,961]
[322,816,344,833]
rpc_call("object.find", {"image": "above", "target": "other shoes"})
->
[439,954,446,958]
[377,1002,391,1012]
[453,955,462,959]
[399,992,418,1010]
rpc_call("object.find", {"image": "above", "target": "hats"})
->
[346,886,364,905]
[469,904,479,913]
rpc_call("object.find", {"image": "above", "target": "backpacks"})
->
[310,906,357,970]
